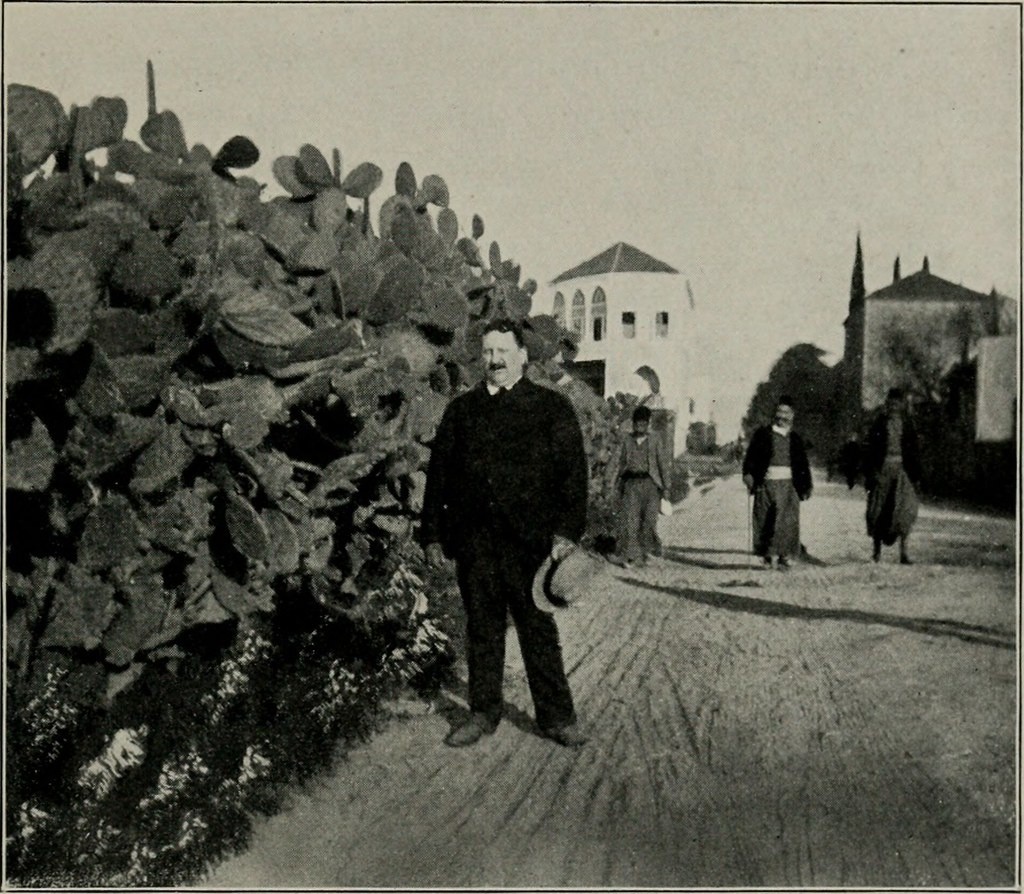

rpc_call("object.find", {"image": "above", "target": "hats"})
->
[634,405,652,420]
[531,535,594,613]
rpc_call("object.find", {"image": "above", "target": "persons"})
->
[421,318,589,748]
[863,388,919,565]
[742,405,814,567]
[616,406,672,567]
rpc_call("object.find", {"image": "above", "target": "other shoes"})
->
[443,713,501,748]
[620,552,660,569]
[543,725,591,748]
[899,558,914,564]
[764,556,794,568]
[871,553,880,563]
